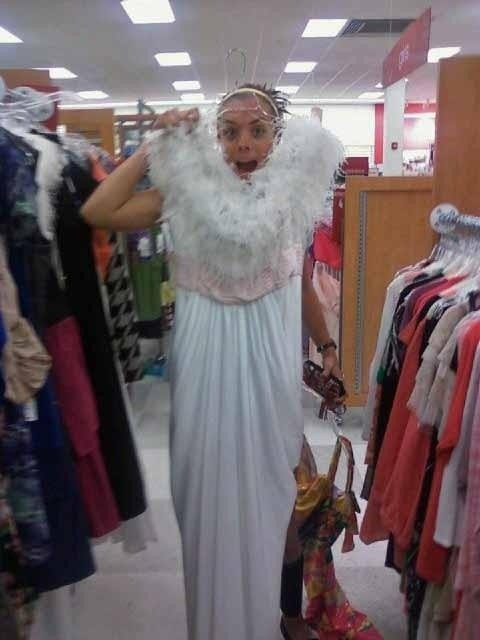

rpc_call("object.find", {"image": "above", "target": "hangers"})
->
[0,79,83,146]
[414,204,480,295]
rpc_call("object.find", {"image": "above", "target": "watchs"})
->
[317,338,337,353]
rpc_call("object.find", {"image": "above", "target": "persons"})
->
[80,83,348,640]
